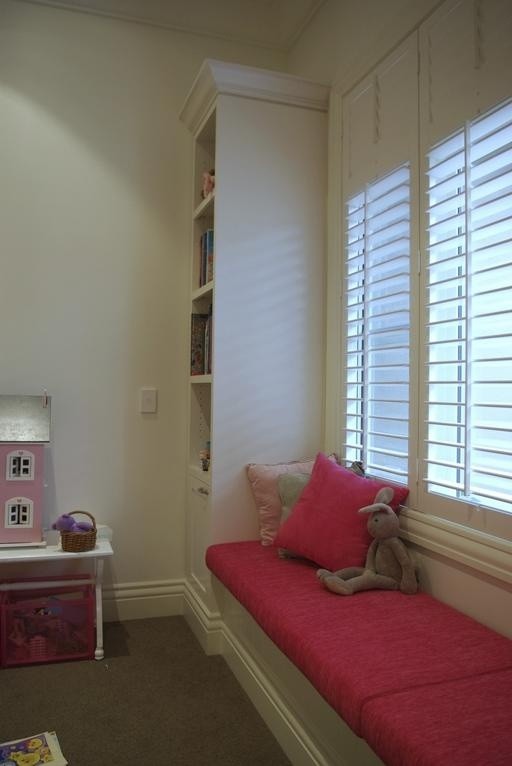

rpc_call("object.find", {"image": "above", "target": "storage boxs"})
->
[1,574,96,670]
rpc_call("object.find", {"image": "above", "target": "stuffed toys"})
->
[317,487,419,596]
[51,514,92,532]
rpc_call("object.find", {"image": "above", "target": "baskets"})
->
[61,511,97,552]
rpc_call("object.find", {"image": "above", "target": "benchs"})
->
[207,526,512,766]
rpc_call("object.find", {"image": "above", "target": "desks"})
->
[1,530,115,668]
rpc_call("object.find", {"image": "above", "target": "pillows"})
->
[242,451,409,570]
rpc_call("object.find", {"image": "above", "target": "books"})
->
[0,730,68,766]
[190,229,213,376]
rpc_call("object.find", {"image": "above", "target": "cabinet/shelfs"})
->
[175,56,332,656]
